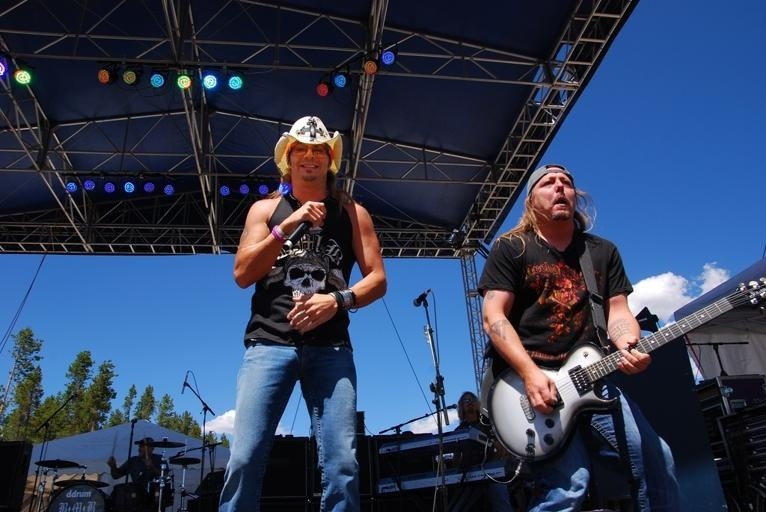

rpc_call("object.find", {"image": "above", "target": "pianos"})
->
[378,427,510,497]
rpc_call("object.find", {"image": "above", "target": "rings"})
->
[296,302,305,310]
[298,310,306,318]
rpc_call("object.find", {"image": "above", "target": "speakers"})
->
[0,441,33,512]
[611,335,703,470]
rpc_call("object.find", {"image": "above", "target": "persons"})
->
[476,164,682,512]
[213,115,388,511]
[453,391,516,512]
[105,437,169,510]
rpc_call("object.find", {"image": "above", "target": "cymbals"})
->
[143,441,185,447]
[170,458,201,465]
[55,480,109,488]
[35,460,78,469]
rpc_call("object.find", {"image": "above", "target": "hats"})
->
[274,116,343,177]
[526,164,574,195]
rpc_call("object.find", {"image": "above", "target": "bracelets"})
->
[271,224,289,243]
[329,289,356,312]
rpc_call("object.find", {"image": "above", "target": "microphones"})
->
[283,221,313,249]
[446,404,456,409]
[181,372,190,394]
[413,289,431,307]
[71,394,78,398]
[130,418,137,424]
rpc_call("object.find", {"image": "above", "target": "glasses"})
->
[463,397,476,403]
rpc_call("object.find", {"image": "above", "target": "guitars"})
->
[487,275,766,461]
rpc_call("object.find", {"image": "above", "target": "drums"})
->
[113,482,149,511]
[47,485,114,511]
